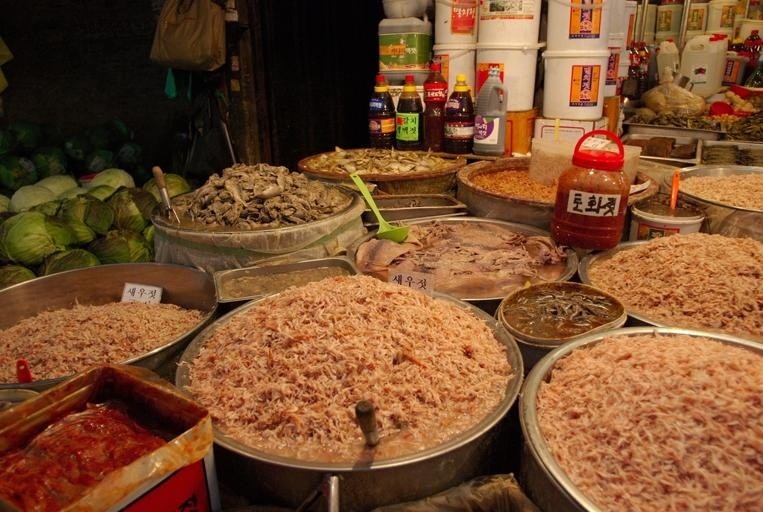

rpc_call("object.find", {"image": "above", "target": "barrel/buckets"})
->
[626,194,704,242]
[428,0,639,155]
[492,280,628,377]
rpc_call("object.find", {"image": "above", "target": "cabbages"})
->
[0,168,191,290]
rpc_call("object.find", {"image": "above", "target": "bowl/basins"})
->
[577,238,670,327]
[662,164,763,213]
[518,326,762,511]
[344,215,576,303]
[0,263,219,390]
[170,286,524,474]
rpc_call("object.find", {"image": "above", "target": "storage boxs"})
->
[533,118,609,146]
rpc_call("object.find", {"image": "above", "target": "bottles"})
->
[722,28,763,85]
[368,62,473,149]
[620,39,650,97]
